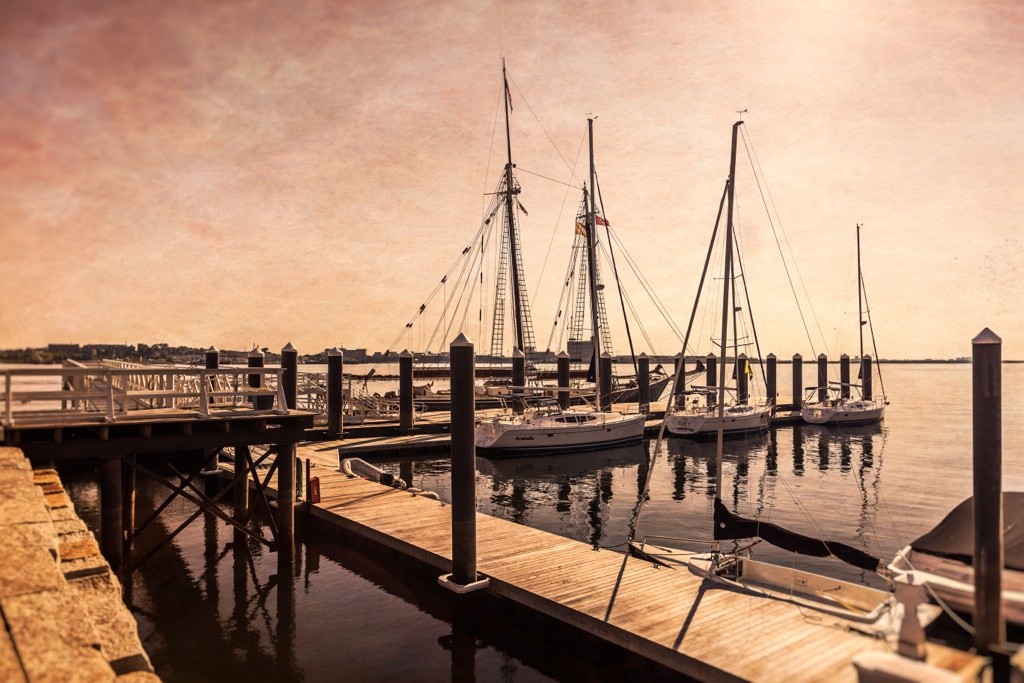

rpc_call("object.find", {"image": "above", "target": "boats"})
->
[882,486,1024,630]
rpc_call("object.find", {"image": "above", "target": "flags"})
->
[745,361,754,380]
[575,222,587,237]
[595,214,609,226]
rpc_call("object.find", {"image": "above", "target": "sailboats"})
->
[799,222,890,428]
[662,181,776,441]
[618,118,946,645]
[468,57,649,452]
